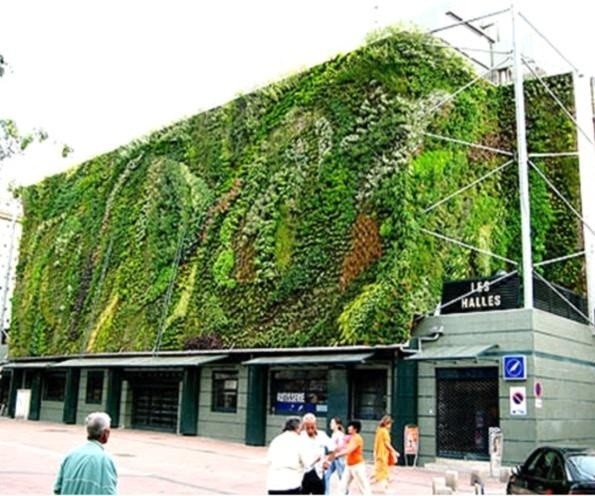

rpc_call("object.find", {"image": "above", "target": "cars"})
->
[505,442,595,495]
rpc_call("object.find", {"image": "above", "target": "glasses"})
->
[87,435,101,440]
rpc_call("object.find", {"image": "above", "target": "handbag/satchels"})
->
[389,451,398,466]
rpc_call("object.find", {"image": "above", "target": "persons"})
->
[325,416,372,495]
[475,430,486,449]
[370,416,400,487]
[267,416,321,494]
[298,413,338,494]
[55,413,117,495]
[491,437,501,460]
